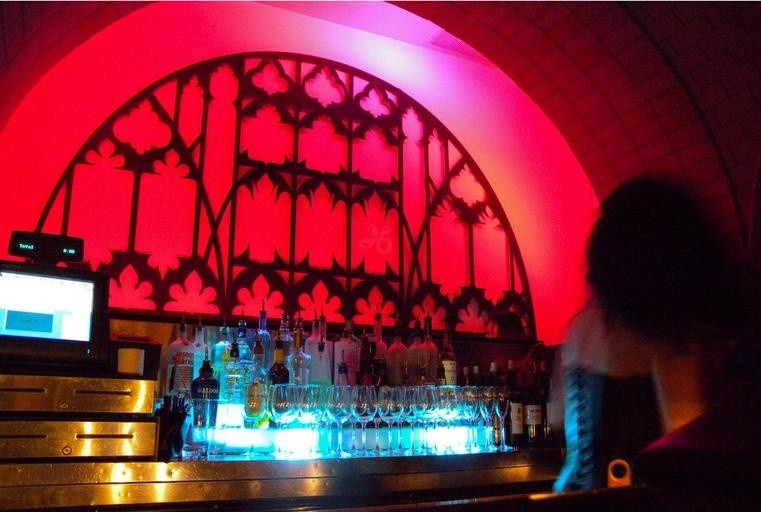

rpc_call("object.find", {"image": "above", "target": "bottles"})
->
[156,310,564,462]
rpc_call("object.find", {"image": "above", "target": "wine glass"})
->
[232,382,519,460]
